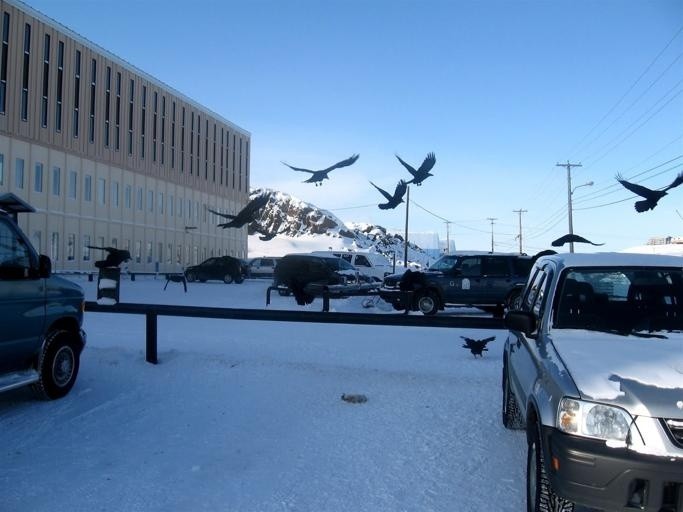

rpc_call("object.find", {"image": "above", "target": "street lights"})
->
[0,193,35,264]
[568,181,594,252]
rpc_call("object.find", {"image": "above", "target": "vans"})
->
[0,211,87,401]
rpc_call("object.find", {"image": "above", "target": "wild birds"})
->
[281,154,361,186]
[208,192,273,229]
[552,234,605,246]
[459,336,495,359]
[396,154,436,185]
[615,170,683,212]
[368,180,407,209]
[252,227,291,241]
[83,246,132,268]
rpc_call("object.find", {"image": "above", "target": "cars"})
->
[503,252,683,512]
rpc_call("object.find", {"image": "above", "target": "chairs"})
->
[560,276,677,332]
